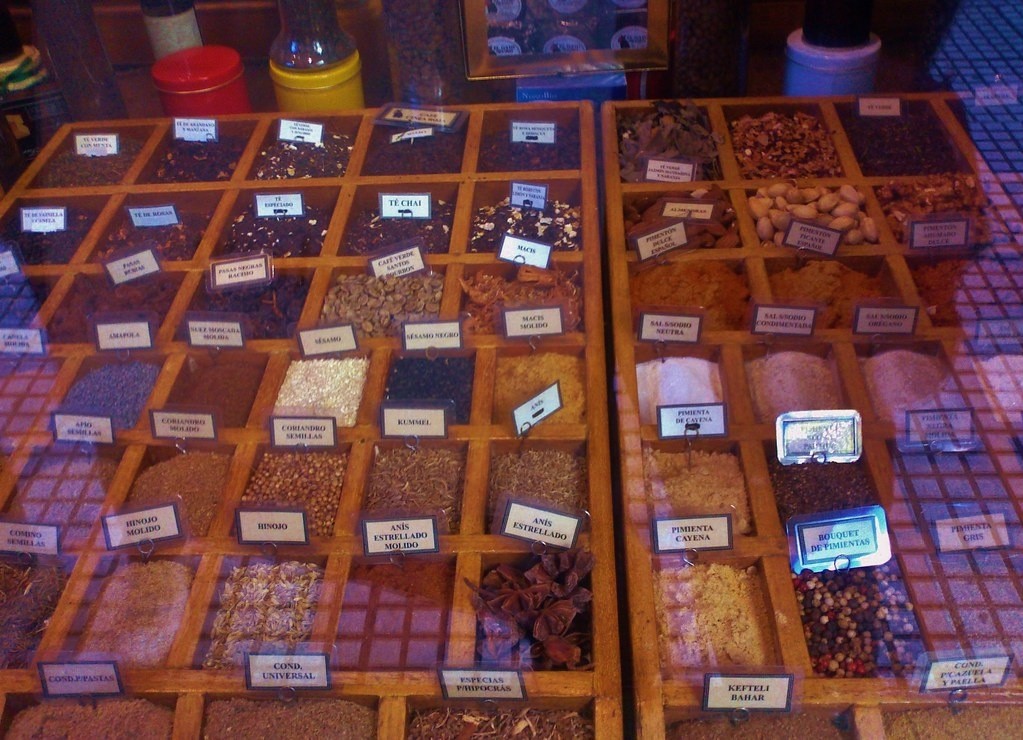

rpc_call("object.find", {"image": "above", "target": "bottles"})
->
[269,0,358,72]
[140,0,204,60]
